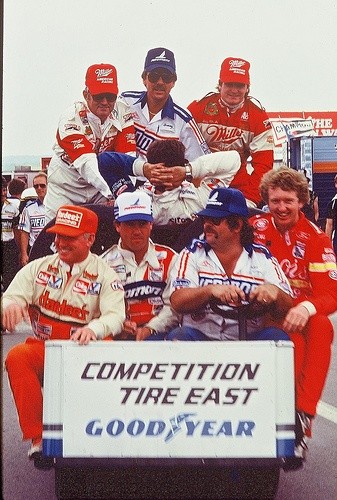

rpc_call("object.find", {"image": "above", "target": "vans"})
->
[2,170,45,198]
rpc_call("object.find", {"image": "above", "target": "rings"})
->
[263,298,267,302]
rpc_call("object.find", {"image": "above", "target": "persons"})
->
[0,203,127,459]
[98,138,242,254]
[296,169,318,222]
[7,179,25,208]
[187,57,274,208]
[42,63,139,226]
[1,177,19,335]
[119,47,210,164]
[98,189,179,342]
[248,164,337,460]
[324,174,337,260]
[168,188,295,342]
[19,173,54,265]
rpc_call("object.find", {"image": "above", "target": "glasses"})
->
[87,90,118,102]
[147,71,175,84]
[201,216,225,226]
[33,183,47,189]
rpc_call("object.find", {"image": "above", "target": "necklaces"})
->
[150,109,158,117]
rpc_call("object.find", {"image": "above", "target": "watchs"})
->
[185,166,194,182]
[150,329,156,335]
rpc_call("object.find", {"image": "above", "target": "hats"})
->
[144,47,176,74]
[114,192,154,222]
[195,188,249,219]
[46,205,99,237]
[85,63,119,95]
[220,57,251,84]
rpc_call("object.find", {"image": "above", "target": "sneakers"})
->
[294,411,312,460]
[27,438,42,460]
